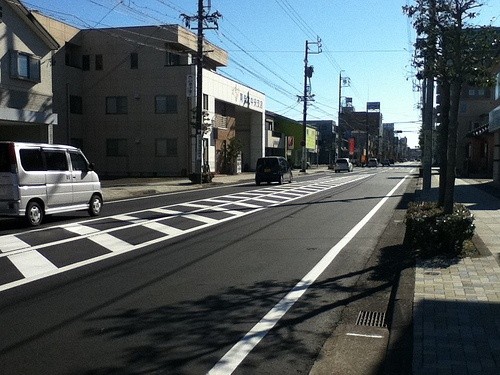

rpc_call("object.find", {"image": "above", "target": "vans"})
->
[0,141,104,228]
[255,156,294,186]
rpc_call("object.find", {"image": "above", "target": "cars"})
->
[368,158,378,168]
[383,158,395,167]
[335,158,354,174]
